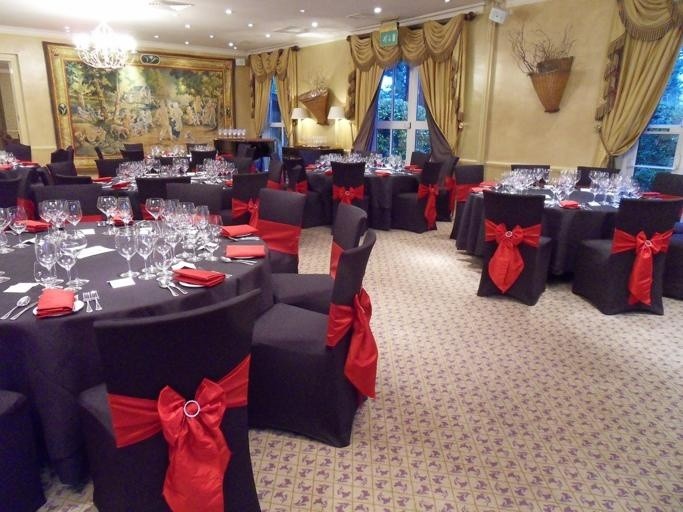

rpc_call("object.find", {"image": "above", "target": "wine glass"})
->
[318,151,404,172]
[150,143,186,157]
[495,167,644,206]
[217,127,245,140]
[0,195,226,292]
[114,158,238,183]
[0,150,18,170]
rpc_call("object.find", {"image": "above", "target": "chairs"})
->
[0,386,46,510]
[0,135,682,317]
[247,230,377,446]
[78,286,264,510]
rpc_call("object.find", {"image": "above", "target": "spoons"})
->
[158,278,178,297]
[0,296,31,319]
[227,236,262,242]
[219,256,257,264]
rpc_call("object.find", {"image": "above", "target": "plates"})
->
[32,299,84,317]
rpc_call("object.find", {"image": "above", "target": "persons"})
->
[77,94,218,145]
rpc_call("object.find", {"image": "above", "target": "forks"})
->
[83,289,103,313]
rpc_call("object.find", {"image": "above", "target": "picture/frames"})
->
[42,40,236,173]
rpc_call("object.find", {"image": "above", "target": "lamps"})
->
[291,108,311,147]
[69,24,139,70]
[327,106,347,148]
